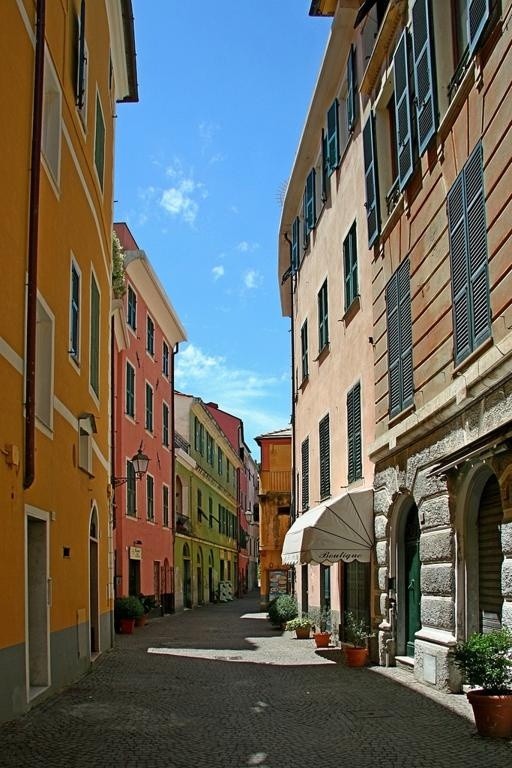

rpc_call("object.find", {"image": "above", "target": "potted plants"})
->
[115,594,160,634]
[445,624,512,738]
[267,593,377,668]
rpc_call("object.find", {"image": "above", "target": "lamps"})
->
[114,449,152,489]
